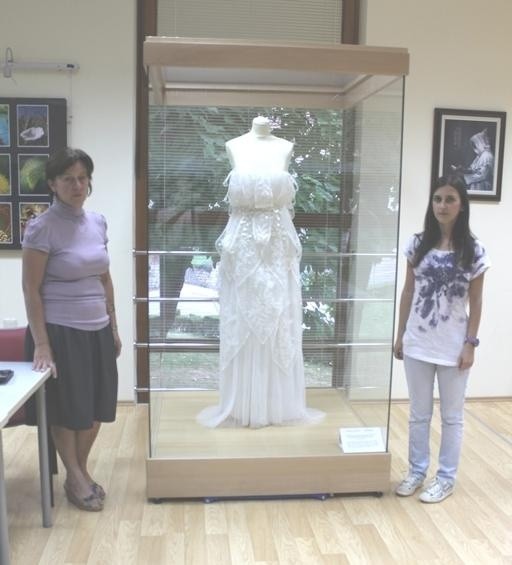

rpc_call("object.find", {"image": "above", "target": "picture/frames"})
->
[0,96,68,251]
[430,108,506,203]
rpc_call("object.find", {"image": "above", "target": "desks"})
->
[0,359,54,565]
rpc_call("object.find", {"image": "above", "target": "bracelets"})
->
[33,342,49,347]
[466,336,480,348]
[112,326,120,335]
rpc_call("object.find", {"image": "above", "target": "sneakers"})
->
[395,474,424,496]
[419,477,455,503]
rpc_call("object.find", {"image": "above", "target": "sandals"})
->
[63,481,104,512]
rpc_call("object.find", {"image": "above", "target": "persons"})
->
[21,147,122,514]
[224,114,297,428]
[447,124,494,193]
[391,176,491,505]
